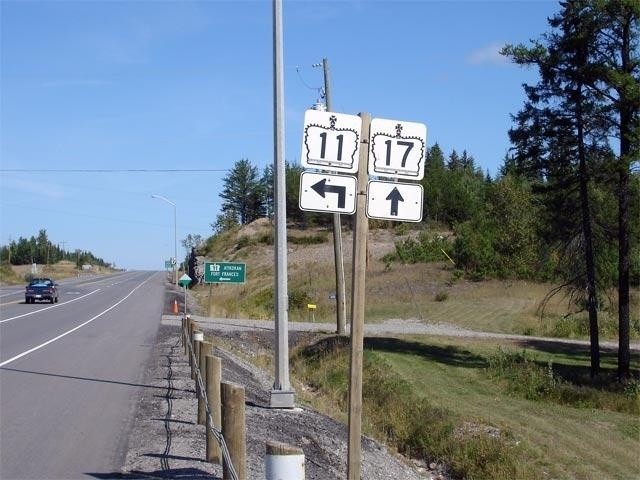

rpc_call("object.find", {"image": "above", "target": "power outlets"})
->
[25,277,58,304]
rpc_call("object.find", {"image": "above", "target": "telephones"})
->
[204,262,246,284]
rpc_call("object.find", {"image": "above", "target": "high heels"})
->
[172,298,180,314]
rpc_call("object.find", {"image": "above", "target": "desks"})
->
[151,194,179,284]
